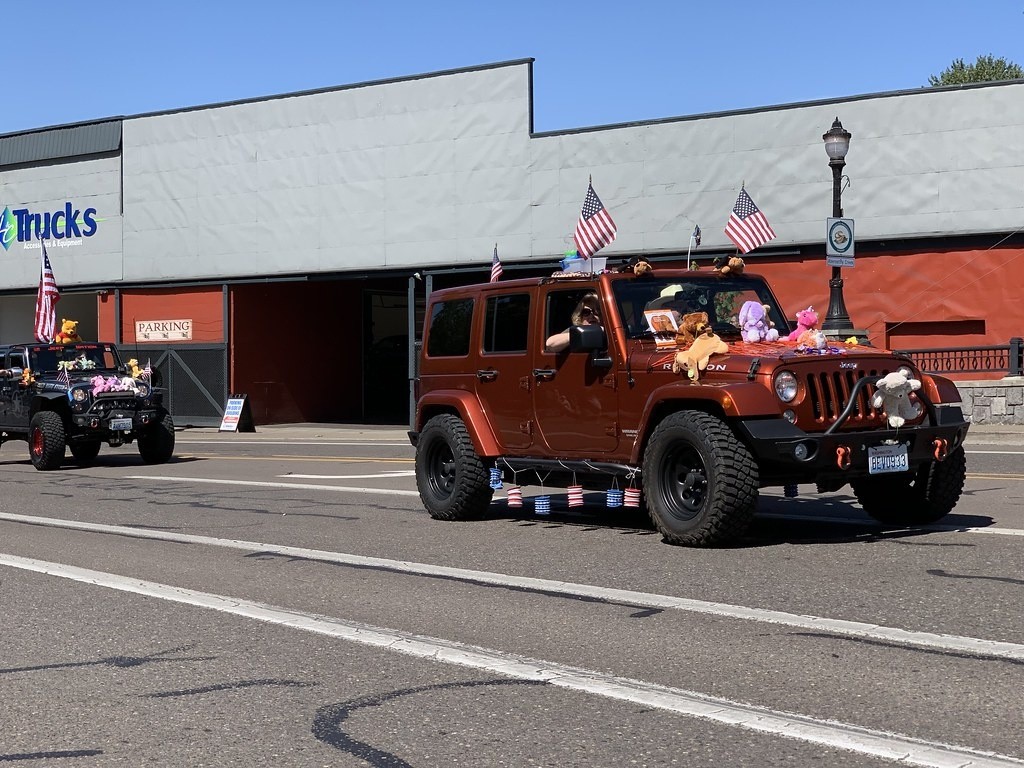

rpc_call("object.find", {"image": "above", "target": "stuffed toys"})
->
[53,319,83,344]
[796,328,829,350]
[738,301,780,343]
[90,375,141,396]
[56,353,96,370]
[676,327,729,381]
[675,312,709,349]
[870,368,923,428]
[127,358,144,379]
[19,367,36,385]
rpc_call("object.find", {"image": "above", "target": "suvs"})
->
[406,263,974,549]
[0,337,175,473]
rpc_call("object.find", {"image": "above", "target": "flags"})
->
[142,358,153,380]
[491,242,503,285]
[725,180,777,255]
[780,305,820,341]
[33,232,62,344]
[574,172,618,261]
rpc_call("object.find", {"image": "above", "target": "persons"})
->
[545,292,605,354]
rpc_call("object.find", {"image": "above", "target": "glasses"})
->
[582,308,600,317]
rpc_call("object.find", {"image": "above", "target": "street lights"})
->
[819,114,857,332]
